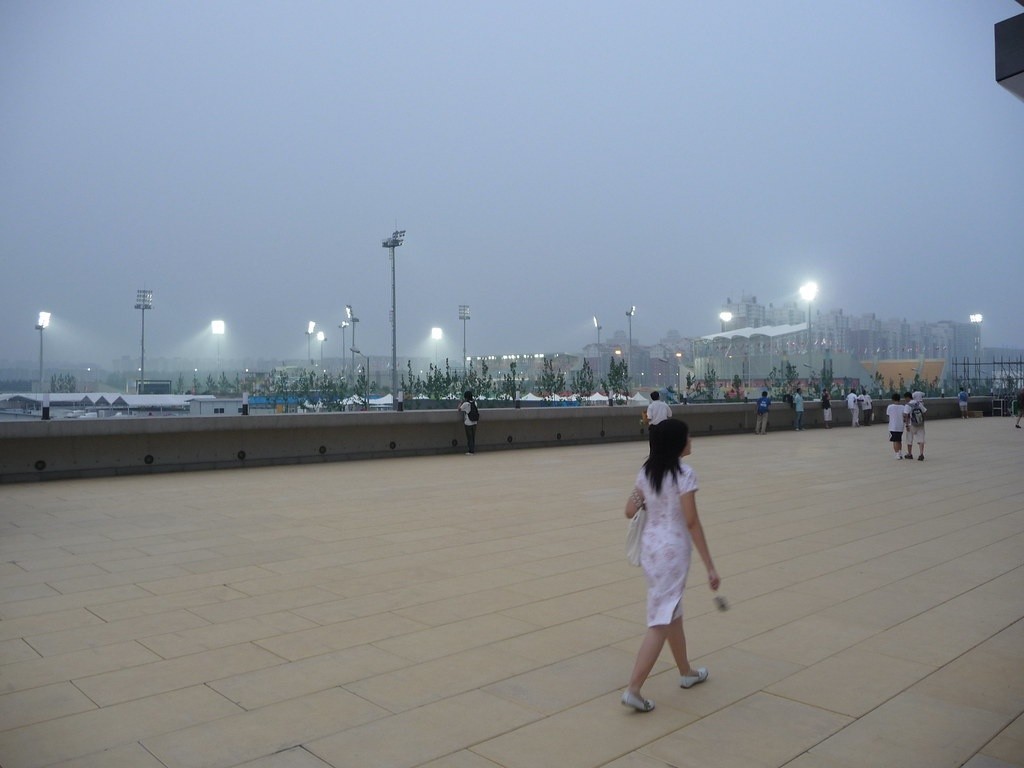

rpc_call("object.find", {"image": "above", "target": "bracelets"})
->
[905,426,909,427]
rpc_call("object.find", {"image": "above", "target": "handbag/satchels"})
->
[624,504,647,569]
[755,398,761,415]
[783,394,795,403]
[870,412,874,421]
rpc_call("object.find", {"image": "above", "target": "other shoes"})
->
[904,454,913,459]
[465,451,474,456]
[917,455,924,461]
[1016,425,1021,428]
[897,455,903,460]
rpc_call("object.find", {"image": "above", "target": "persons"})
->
[793,388,805,431]
[887,394,910,459]
[958,388,968,418]
[755,391,771,435]
[904,392,928,461]
[846,389,859,427]
[623,419,720,711]
[912,388,925,407]
[458,391,478,455]
[645,392,672,454]
[822,388,832,429]
[857,389,873,426]
[1015,387,1024,428]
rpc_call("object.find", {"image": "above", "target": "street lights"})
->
[969,313,983,357]
[338,319,350,374]
[316,330,328,369]
[458,304,471,378]
[625,305,638,372]
[134,289,154,393]
[345,302,360,372]
[431,327,444,362]
[383,230,406,402]
[34,311,52,392]
[798,280,819,377]
[211,320,225,371]
[304,320,316,360]
[592,312,603,357]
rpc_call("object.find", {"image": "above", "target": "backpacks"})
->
[907,402,925,427]
[959,391,967,402]
[467,401,479,421]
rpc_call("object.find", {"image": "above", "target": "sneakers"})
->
[679,667,707,689]
[622,690,655,712]
